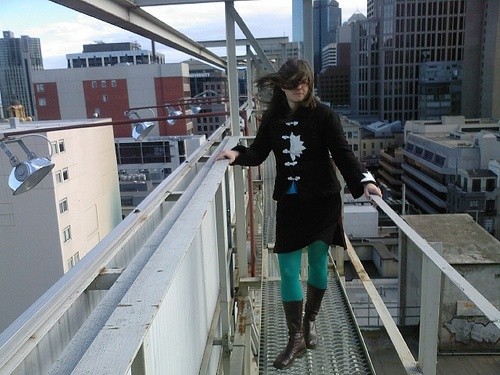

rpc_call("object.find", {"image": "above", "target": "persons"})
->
[215,57,382,368]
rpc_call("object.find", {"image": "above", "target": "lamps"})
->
[123,110,155,142]
[164,103,184,125]
[0,133,56,195]
[189,104,202,115]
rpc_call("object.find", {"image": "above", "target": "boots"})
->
[273,300,307,370]
[303,283,327,349]
